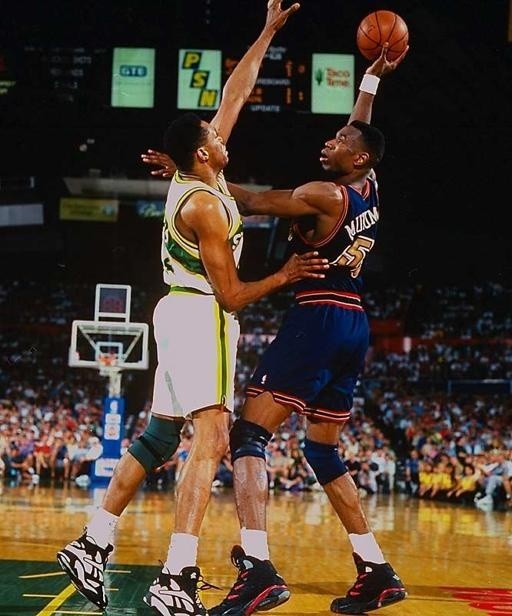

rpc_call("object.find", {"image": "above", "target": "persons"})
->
[57,0,328,616]
[141,41,411,615]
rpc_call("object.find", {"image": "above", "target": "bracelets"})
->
[359,74,381,96]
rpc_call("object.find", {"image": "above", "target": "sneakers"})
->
[142,559,209,616]
[57,527,116,611]
[329,551,409,615]
[207,544,290,615]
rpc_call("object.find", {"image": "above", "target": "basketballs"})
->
[358,10,408,61]
